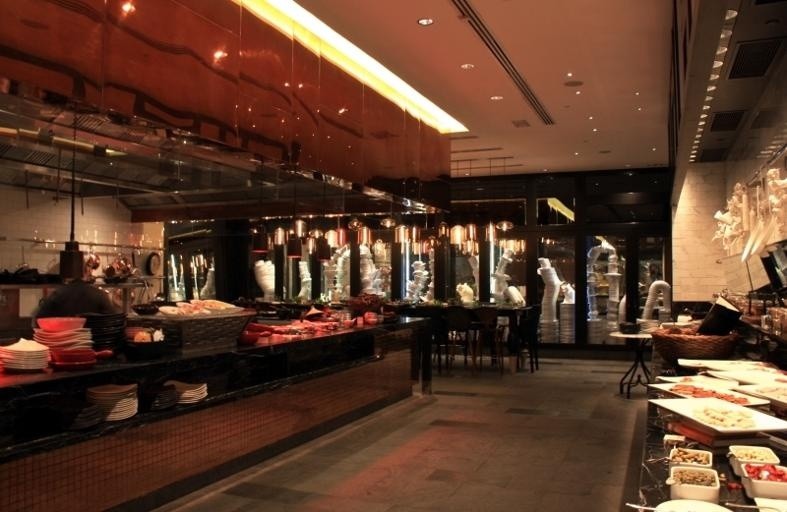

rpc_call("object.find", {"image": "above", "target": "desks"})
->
[609,331,654,399]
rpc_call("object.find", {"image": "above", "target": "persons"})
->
[32,263,115,328]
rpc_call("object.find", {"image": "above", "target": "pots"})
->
[86,242,142,279]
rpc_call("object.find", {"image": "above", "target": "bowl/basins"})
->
[132,301,176,316]
[239,331,259,346]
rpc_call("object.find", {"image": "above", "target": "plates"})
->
[619,318,693,334]
[645,358,787,435]
[1,317,209,423]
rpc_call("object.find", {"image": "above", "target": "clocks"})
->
[146,252,160,275]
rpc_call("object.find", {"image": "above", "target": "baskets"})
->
[652,330,739,366]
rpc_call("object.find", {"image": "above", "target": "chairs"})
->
[234,300,542,375]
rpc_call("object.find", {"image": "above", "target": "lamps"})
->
[59,79,84,278]
[246,210,526,257]
